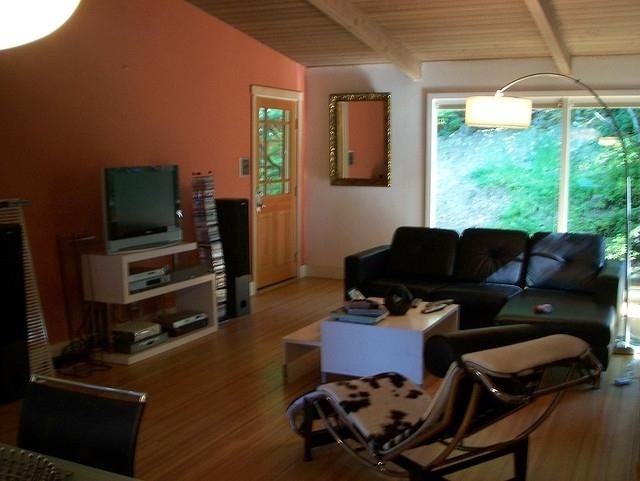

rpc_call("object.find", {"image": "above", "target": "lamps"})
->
[461,70,640,355]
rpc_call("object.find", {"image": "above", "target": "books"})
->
[330,306,390,324]
[192,174,228,319]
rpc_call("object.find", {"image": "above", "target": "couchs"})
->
[340,225,628,393]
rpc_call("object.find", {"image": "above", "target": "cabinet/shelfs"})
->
[72,238,221,370]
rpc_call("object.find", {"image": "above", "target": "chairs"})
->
[280,322,609,480]
[15,371,150,480]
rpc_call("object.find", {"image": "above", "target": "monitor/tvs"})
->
[100,163,183,256]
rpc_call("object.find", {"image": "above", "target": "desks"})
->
[1,442,75,481]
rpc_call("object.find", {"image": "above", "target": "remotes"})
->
[411,298,422,308]
[426,299,455,307]
[423,304,447,314]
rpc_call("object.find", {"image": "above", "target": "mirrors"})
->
[325,89,394,189]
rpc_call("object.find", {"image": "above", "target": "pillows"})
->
[418,322,548,381]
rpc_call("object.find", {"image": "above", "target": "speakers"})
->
[0,224,30,405]
[214,197,249,317]
